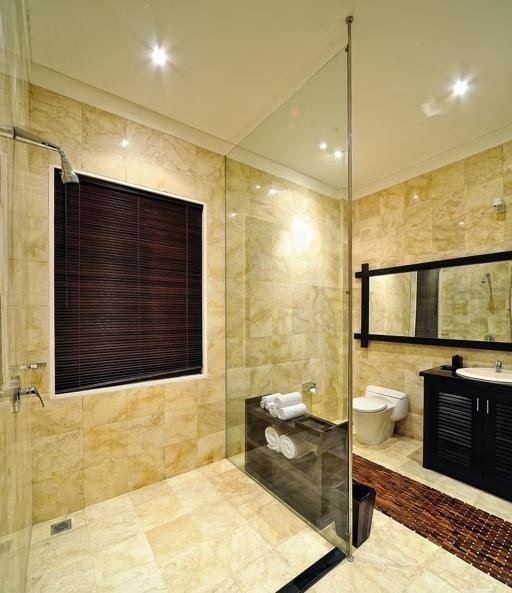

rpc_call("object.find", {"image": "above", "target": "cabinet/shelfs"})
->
[242,392,350,532]
[415,367,512,502]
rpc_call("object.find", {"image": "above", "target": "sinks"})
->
[455,367,512,386]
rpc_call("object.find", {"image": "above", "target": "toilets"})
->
[352,385,409,446]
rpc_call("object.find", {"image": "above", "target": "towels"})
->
[262,424,286,451]
[278,429,317,460]
[257,391,308,420]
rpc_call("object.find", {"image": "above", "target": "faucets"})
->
[495,360,503,372]
[484,335,496,342]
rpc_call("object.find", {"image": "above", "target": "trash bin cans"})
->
[335,478,377,547]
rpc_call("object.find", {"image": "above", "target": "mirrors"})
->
[354,250,512,350]
